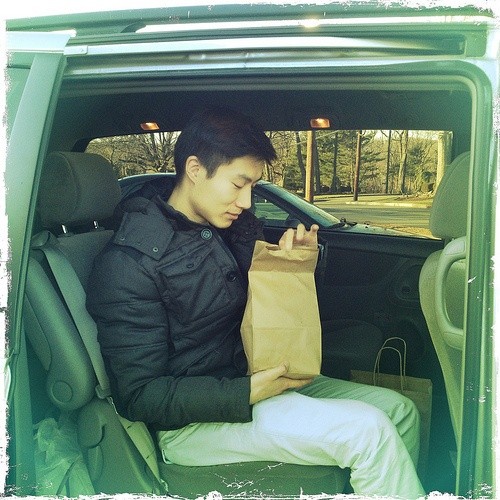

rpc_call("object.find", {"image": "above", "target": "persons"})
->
[85,108,425,497]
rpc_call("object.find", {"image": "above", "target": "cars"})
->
[117,171,428,239]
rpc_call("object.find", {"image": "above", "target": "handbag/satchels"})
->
[352,337,433,459]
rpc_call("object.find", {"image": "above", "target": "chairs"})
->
[35,152,353,496]
